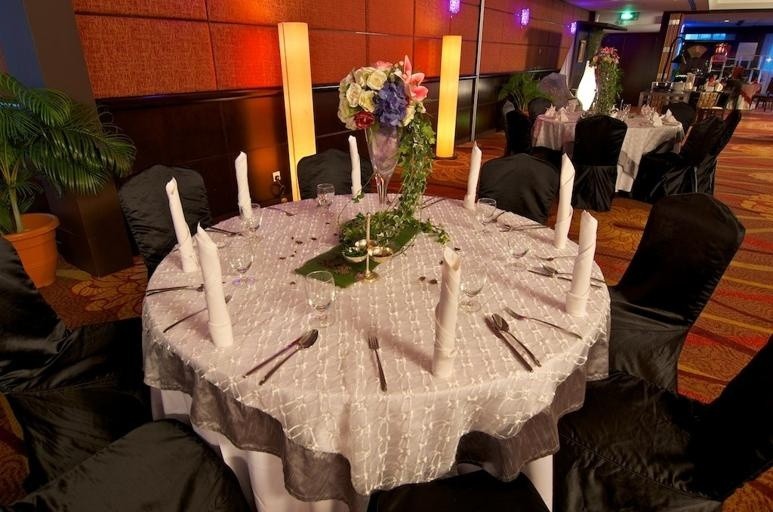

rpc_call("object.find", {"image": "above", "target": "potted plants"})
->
[497,73,554,117]
[0,74,138,288]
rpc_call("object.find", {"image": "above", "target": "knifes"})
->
[527,269,600,291]
[484,314,533,373]
[243,334,302,379]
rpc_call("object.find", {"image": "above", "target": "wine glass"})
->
[461,263,485,312]
[318,184,335,224]
[241,204,263,244]
[227,240,256,289]
[508,231,528,272]
[307,271,336,327]
[477,199,495,237]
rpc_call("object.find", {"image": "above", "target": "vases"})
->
[363,126,401,221]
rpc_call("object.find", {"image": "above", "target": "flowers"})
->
[591,47,618,110]
[336,55,428,170]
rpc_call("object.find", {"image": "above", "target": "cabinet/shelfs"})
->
[708,55,762,83]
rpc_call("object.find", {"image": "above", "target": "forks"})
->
[535,253,580,261]
[548,101,673,128]
[368,333,389,391]
[163,287,236,334]
[504,306,583,340]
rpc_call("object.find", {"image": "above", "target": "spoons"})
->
[543,266,606,285]
[259,329,320,388]
[492,312,542,368]
[142,283,204,297]
[485,211,551,232]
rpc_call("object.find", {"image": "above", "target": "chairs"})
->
[504,80,773,211]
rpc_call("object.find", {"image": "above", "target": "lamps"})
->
[277,21,317,200]
[436,35,462,157]
[661,37,687,82]
